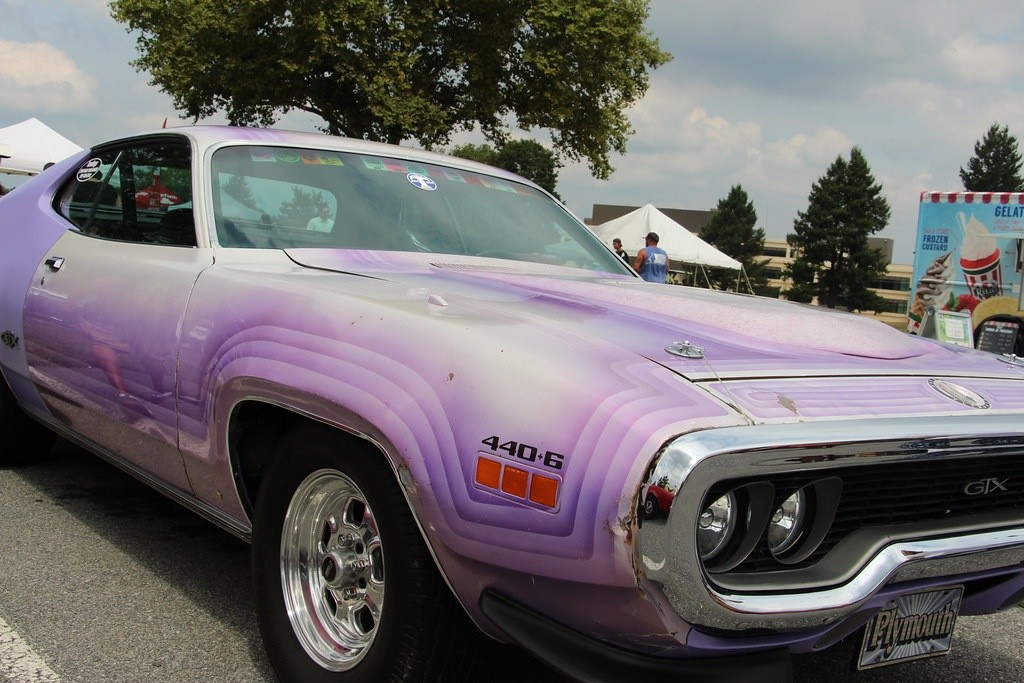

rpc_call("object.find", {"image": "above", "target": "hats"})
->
[643,233,659,242]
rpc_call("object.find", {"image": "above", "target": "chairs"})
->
[156,207,251,249]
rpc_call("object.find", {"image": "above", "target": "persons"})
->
[630,232,670,284]
[262,213,278,231]
[612,239,629,263]
[306,206,333,232]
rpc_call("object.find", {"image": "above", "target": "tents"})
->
[545,203,743,275]
[0,117,87,177]
[136,183,178,208]
[168,185,268,229]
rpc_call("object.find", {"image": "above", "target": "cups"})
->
[960,246,1003,302]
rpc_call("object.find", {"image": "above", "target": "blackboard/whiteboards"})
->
[976,320,1019,355]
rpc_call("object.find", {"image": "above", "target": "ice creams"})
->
[905,251,954,336]
[959,212,1003,301]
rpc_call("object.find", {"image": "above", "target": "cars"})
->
[0,124,1023,683]
